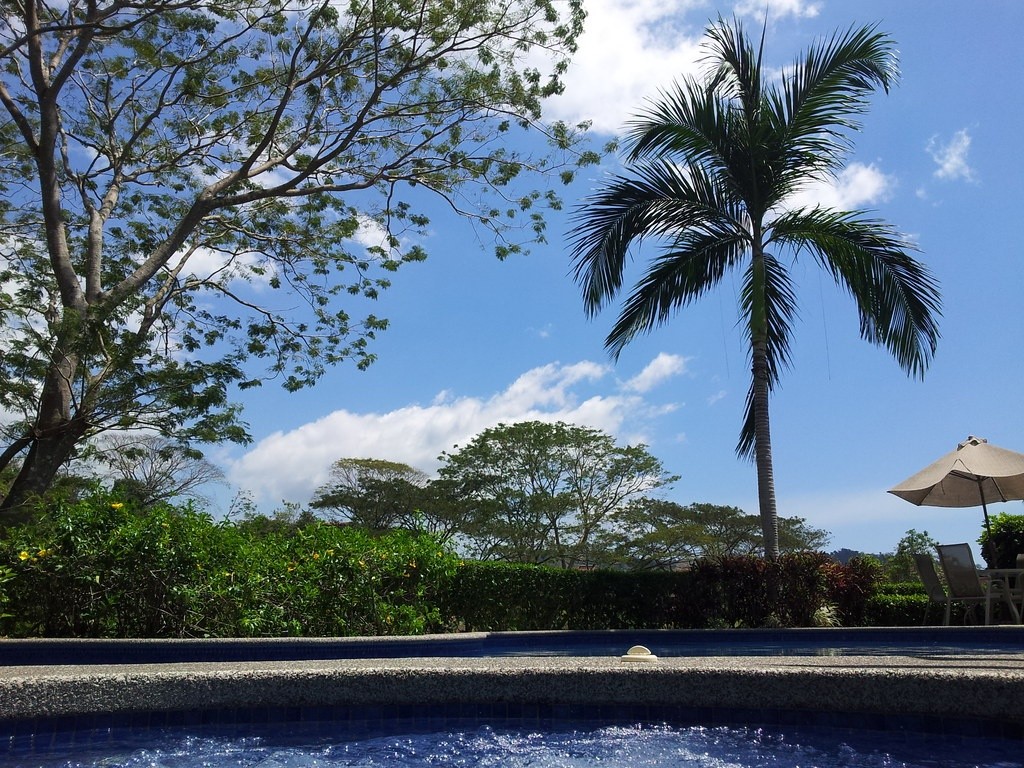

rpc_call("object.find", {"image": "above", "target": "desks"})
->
[964,569,1024,626]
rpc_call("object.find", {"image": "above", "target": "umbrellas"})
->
[886,435,1024,568]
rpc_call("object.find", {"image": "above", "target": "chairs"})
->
[934,543,1022,624]
[988,554,1024,625]
[911,553,993,626]
